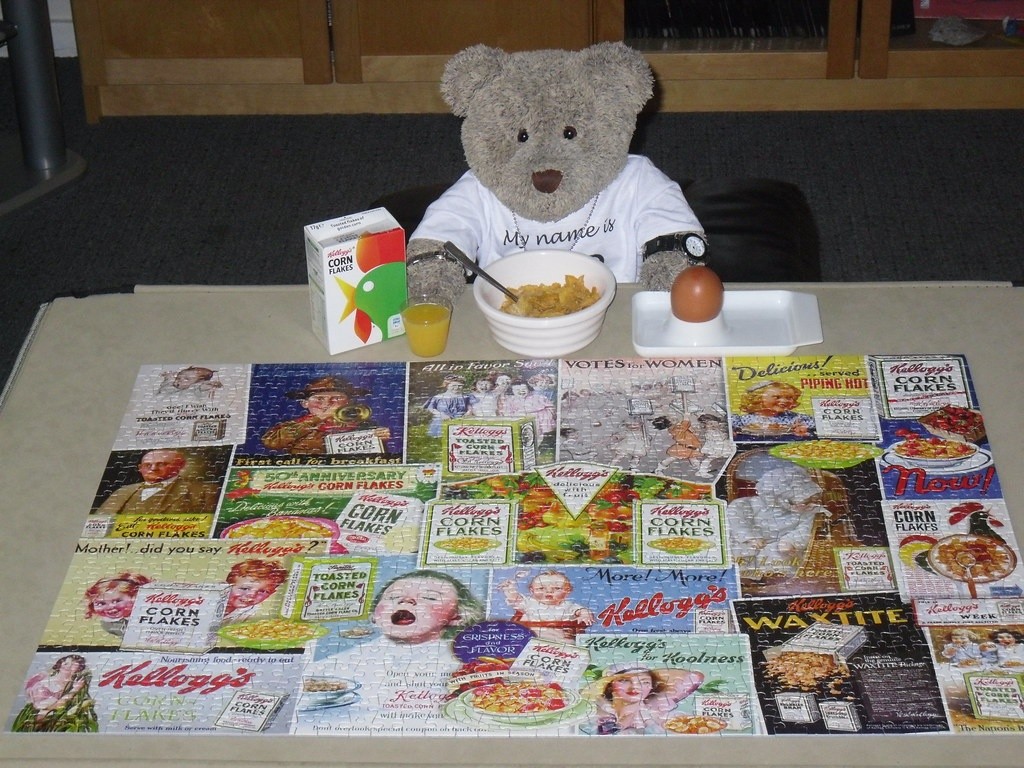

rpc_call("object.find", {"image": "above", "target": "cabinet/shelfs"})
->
[72,0,1024,124]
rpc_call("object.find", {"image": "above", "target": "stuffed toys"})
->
[405,40,709,310]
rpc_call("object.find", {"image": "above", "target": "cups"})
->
[399,295,453,358]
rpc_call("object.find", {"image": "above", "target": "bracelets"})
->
[406,250,467,279]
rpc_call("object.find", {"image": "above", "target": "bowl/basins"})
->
[769,442,882,469]
[219,515,340,549]
[218,616,328,650]
[458,682,582,723]
[304,675,362,702]
[473,249,617,358]
[888,438,978,467]
[432,537,503,555]
[647,537,716,555]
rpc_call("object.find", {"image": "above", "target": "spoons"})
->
[956,551,977,599]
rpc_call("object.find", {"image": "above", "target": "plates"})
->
[442,698,598,730]
[876,449,994,474]
[630,289,823,358]
[927,535,1019,584]
[298,692,361,711]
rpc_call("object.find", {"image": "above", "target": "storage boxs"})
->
[304,207,408,355]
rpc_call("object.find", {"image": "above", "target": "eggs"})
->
[670,265,724,323]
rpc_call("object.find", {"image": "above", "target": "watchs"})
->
[643,232,708,265]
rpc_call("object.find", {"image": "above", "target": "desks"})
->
[0,283,1024,768]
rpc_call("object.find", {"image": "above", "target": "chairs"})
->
[370,177,824,283]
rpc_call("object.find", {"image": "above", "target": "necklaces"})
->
[511,192,599,252]
